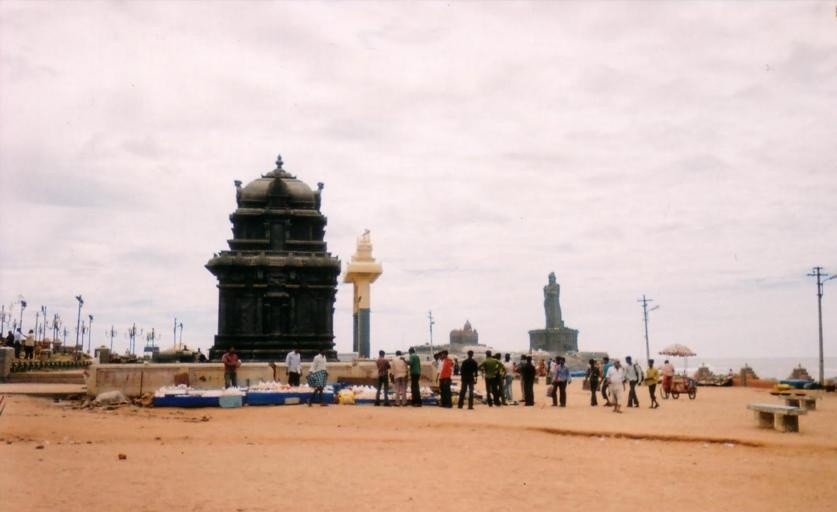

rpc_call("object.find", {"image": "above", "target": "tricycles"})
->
[660,376,696,400]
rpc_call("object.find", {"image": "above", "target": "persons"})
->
[582,356,673,413]
[375,347,573,408]
[268,359,277,382]
[306,347,328,407]
[285,346,303,387]
[221,345,241,389]
[463,320,472,334]
[727,368,734,386]
[0,329,35,359]
[194,348,201,363]
[543,271,562,331]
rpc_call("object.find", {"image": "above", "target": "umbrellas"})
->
[658,343,697,374]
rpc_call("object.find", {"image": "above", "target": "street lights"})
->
[815,274,836,387]
[426,310,434,360]
[643,304,660,364]
[0,295,184,358]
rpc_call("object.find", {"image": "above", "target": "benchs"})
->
[747,382,823,440]
